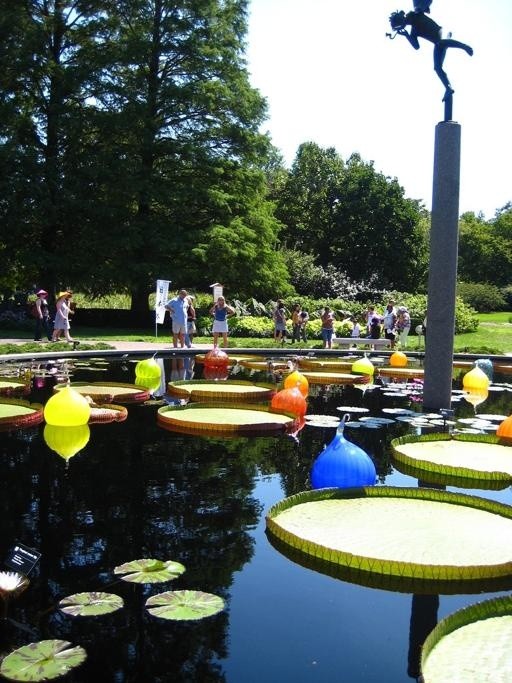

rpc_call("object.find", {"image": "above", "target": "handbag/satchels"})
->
[282,319,287,326]
[31,298,45,320]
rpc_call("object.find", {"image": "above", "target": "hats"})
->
[36,289,47,294]
[56,291,70,300]
[398,306,408,313]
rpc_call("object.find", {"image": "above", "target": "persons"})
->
[422,310,427,337]
[390,10,474,101]
[170,354,195,408]
[34,290,78,342]
[209,296,235,349]
[164,290,197,348]
[273,300,412,350]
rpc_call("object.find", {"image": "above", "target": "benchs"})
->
[331,338,391,351]
